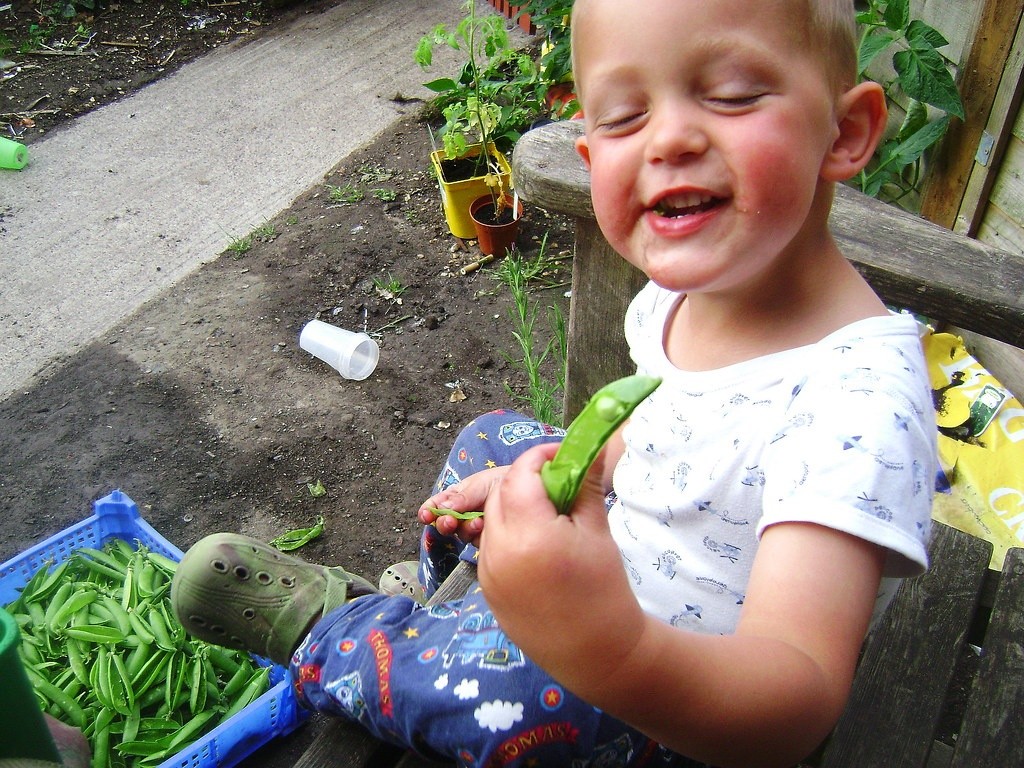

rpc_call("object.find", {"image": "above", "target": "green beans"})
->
[3,536,274,768]
[540,374,663,517]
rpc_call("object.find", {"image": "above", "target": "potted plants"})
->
[414,0,555,258]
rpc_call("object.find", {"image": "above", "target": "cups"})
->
[298,318,380,382]
[0,136,29,169]
[0,607,64,768]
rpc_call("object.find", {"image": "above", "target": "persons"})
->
[171,0,937,767]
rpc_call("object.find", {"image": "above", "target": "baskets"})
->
[0,488,310,768]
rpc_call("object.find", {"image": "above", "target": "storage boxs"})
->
[0,487,313,768]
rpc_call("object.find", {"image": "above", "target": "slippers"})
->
[171,532,381,671]
[380,559,430,607]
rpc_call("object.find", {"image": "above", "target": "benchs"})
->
[295,115,1024,768]
